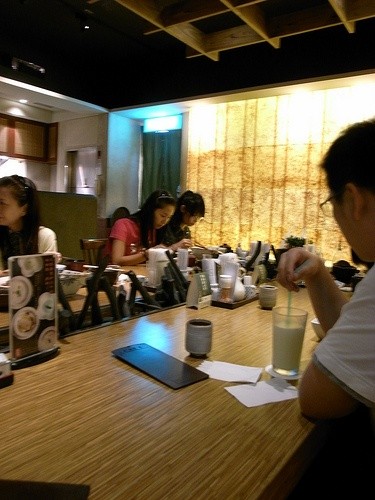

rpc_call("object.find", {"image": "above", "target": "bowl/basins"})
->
[191,244,226,262]
[56,272,92,298]
[90,264,120,284]
[67,258,85,272]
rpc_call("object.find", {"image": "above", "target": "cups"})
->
[55,264,65,276]
[244,284,258,299]
[184,319,213,356]
[311,317,327,340]
[270,306,309,376]
[137,275,146,284]
[258,284,278,308]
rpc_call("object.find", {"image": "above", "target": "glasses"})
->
[319,195,334,217]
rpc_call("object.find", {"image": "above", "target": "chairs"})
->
[80,236,108,264]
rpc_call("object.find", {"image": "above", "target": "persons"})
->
[96,189,177,272]
[0,175,62,277]
[160,190,205,250]
[276,117,375,418]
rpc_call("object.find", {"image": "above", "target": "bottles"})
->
[210,274,232,301]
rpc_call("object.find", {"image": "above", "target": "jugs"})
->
[233,268,246,302]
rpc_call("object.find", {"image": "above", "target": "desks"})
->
[0,281,361,500]
[0,262,201,349]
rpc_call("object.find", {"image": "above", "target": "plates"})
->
[11,276,33,310]
[37,326,55,351]
[18,257,43,277]
[10,307,40,341]
[0,275,10,290]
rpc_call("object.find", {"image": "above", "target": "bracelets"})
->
[143,250,149,260]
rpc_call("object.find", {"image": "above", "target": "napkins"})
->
[224,378,298,408]
[196,360,264,383]
